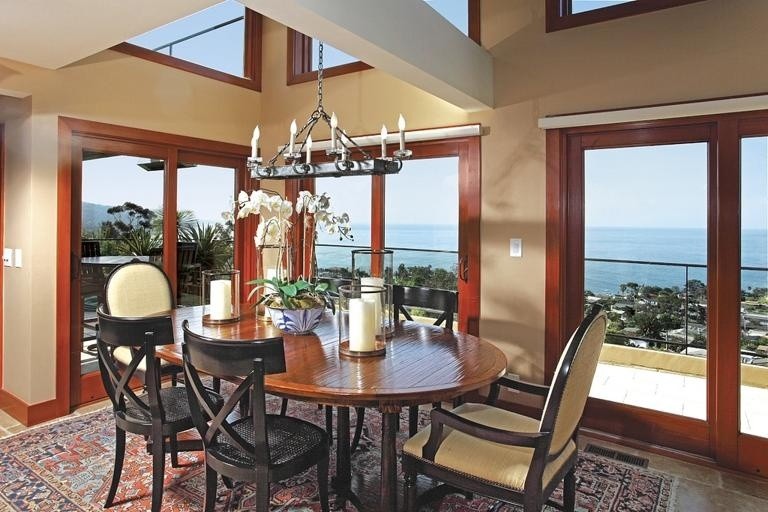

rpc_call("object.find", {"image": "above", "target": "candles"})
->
[359,277,383,336]
[209,280,231,320]
[349,298,374,353]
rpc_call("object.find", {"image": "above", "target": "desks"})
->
[128,300,506,511]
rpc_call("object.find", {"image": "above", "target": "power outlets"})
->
[505,373,520,394]
[2,247,11,267]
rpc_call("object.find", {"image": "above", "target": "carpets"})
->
[0,378,680,511]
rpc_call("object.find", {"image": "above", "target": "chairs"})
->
[143,243,197,306]
[401,305,607,512]
[99,257,176,392]
[80,240,103,310]
[91,304,234,512]
[349,282,458,456]
[179,319,330,511]
[276,275,329,417]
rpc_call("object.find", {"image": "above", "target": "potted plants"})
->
[245,276,329,335]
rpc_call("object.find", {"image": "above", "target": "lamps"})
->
[246,39,412,179]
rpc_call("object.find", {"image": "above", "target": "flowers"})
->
[222,188,354,314]
